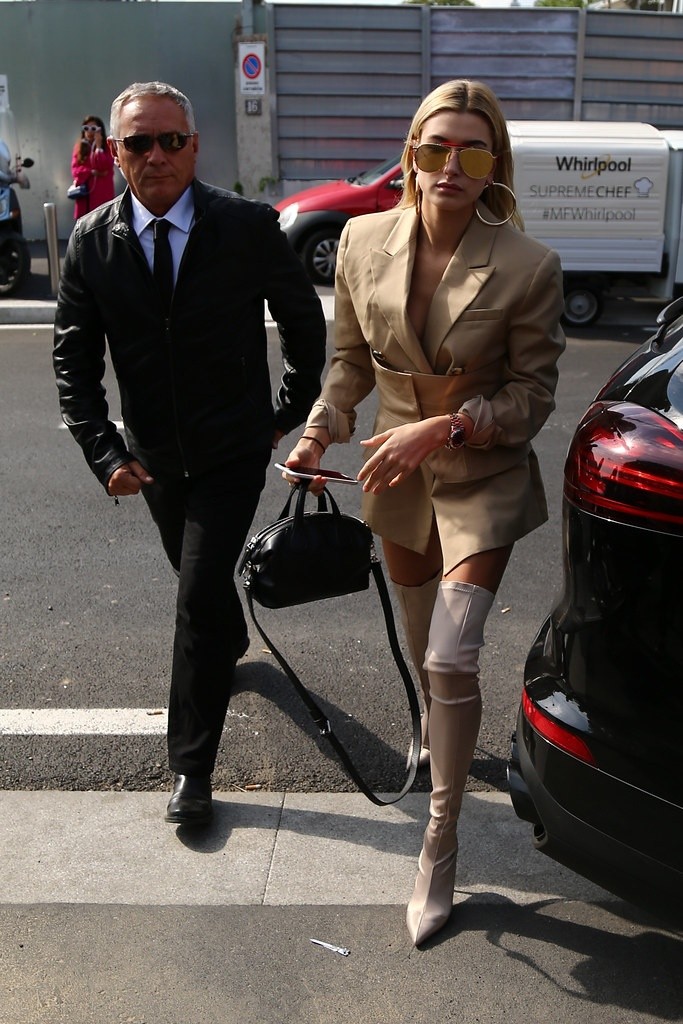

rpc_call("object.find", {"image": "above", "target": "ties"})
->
[150,219,174,319]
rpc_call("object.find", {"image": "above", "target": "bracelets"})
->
[299,434,326,454]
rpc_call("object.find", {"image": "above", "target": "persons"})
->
[50,80,331,824]
[0,140,31,235]
[69,115,117,224]
[281,78,568,948]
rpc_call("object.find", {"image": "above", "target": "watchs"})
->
[444,412,467,452]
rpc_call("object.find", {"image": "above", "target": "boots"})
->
[387,569,443,774]
[405,581,495,947]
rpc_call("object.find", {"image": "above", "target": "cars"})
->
[509,291,683,929]
[272,155,404,288]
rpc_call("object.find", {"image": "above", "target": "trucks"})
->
[499,119,683,330]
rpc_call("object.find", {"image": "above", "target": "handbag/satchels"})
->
[237,479,373,609]
[67,179,88,199]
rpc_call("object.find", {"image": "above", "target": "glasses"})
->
[410,143,499,181]
[81,124,101,133]
[110,131,195,155]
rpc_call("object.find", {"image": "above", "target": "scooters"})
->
[0,142,34,299]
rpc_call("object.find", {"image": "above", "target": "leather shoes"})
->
[165,772,213,823]
[236,636,249,661]
